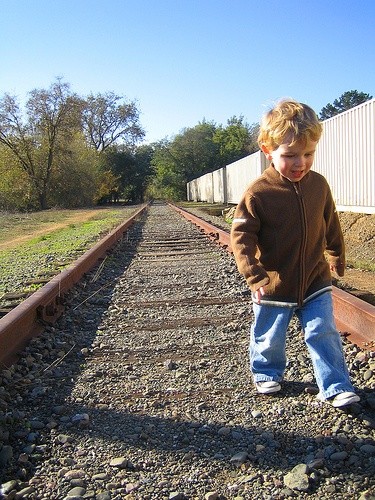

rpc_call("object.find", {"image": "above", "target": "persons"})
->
[229,98,362,408]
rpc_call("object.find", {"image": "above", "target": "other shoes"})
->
[323,392,360,408]
[255,382,280,394]
